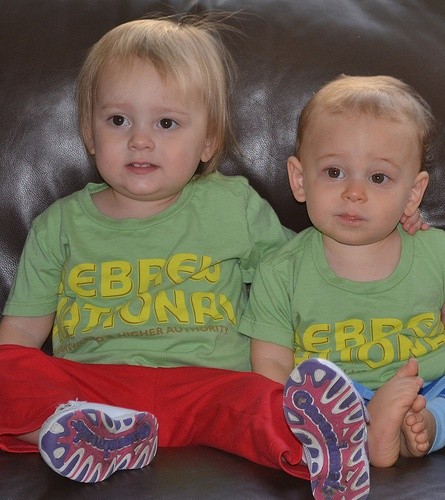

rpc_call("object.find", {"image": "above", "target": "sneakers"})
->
[38,400,158,484]
[283,358,370,500]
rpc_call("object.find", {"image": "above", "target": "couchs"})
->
[0,0,444,500]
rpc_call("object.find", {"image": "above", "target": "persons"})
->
[0,16,372,500]
[249,73,445,471]
